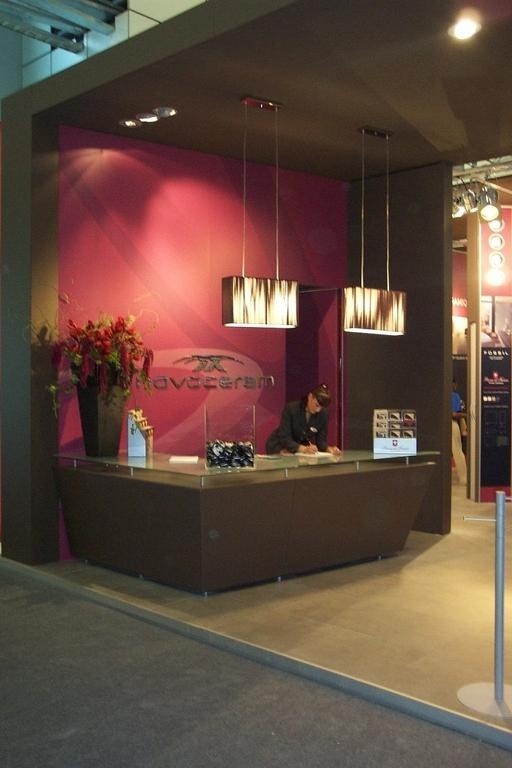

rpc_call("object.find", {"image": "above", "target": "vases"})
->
[74,372,128,457]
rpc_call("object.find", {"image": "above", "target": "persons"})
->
[452,378,467,485]
[267,383,341,456]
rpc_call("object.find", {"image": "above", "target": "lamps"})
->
[343,125,406,336]
[221,93,301,329]
[450,165,506,224]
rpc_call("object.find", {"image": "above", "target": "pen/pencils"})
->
[309,441,319,455]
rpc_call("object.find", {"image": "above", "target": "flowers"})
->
[40,294,154,420]
